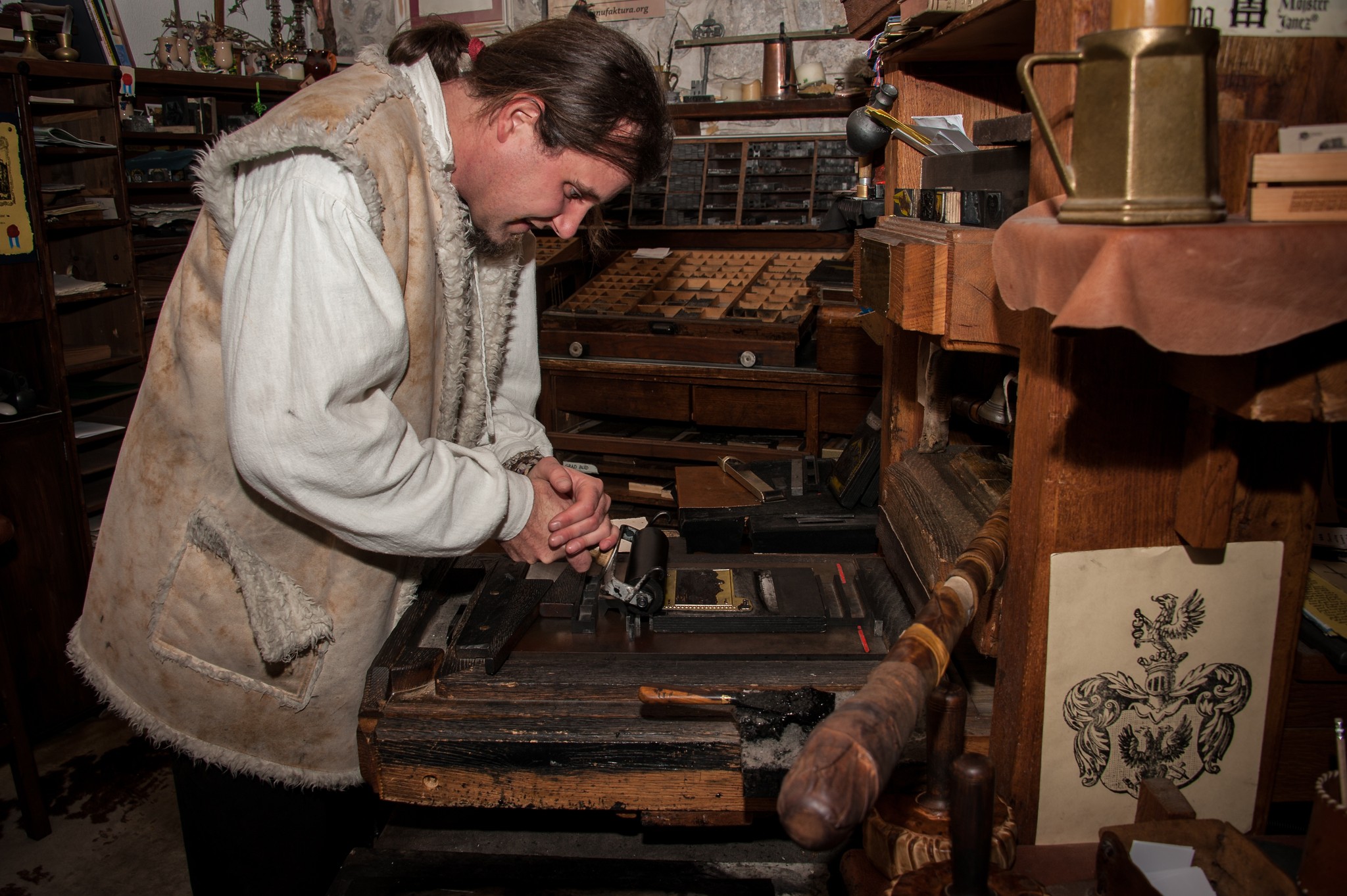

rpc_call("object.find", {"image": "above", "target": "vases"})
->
[157,36,178,69]
[176,39,190,65]
[214,42,233,74]
[54,34,79,62]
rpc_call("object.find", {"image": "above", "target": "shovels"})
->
[638,686,812,715]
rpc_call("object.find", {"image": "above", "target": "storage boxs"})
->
[840,0,901,41]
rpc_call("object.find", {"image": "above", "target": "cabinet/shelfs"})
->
[0,0,1347,896]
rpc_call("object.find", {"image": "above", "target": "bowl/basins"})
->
[665,92,680,103]
[795,62,826,90]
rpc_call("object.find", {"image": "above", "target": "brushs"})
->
[588,511,672,615]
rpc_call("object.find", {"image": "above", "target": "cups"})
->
[762,22,801,100]
[1016,25,1228,224]
[654,71,679,103]
[177,39,190,67]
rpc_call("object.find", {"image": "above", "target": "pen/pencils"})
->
[663,61,670,72]
[103,283,126,287]
[657,47,660,65]
[667,44,673,72]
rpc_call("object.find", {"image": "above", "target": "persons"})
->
[59,21,678,896]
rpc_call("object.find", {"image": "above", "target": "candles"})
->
[21,11,34,31]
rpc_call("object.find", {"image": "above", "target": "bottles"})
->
[118,96,134,123]
[54,33,79,62]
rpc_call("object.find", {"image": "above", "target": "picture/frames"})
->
[393,0,515,37]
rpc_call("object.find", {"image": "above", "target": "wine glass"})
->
[157,37,179,70]
[214,42,233,74]
